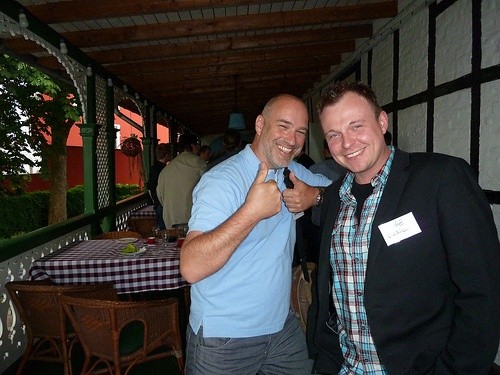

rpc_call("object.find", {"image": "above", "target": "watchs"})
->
[315,187,326,207]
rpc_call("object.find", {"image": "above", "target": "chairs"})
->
[5,281,185,375]
[94,215,184,241]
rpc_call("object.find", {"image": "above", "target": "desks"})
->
[132,205,157,215]
[31,239,190,323]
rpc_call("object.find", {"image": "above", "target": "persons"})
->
[305,80,500,375]
[148,129,349,266]
[180,93,333,375]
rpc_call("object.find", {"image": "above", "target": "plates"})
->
[121,247,147,256]
[115,237,139,243]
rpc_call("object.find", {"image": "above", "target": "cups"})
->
[147,237,155,244]
[177,238,185,248]
[161,230,170,246]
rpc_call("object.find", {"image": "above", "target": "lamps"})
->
[228,74,247,130]
